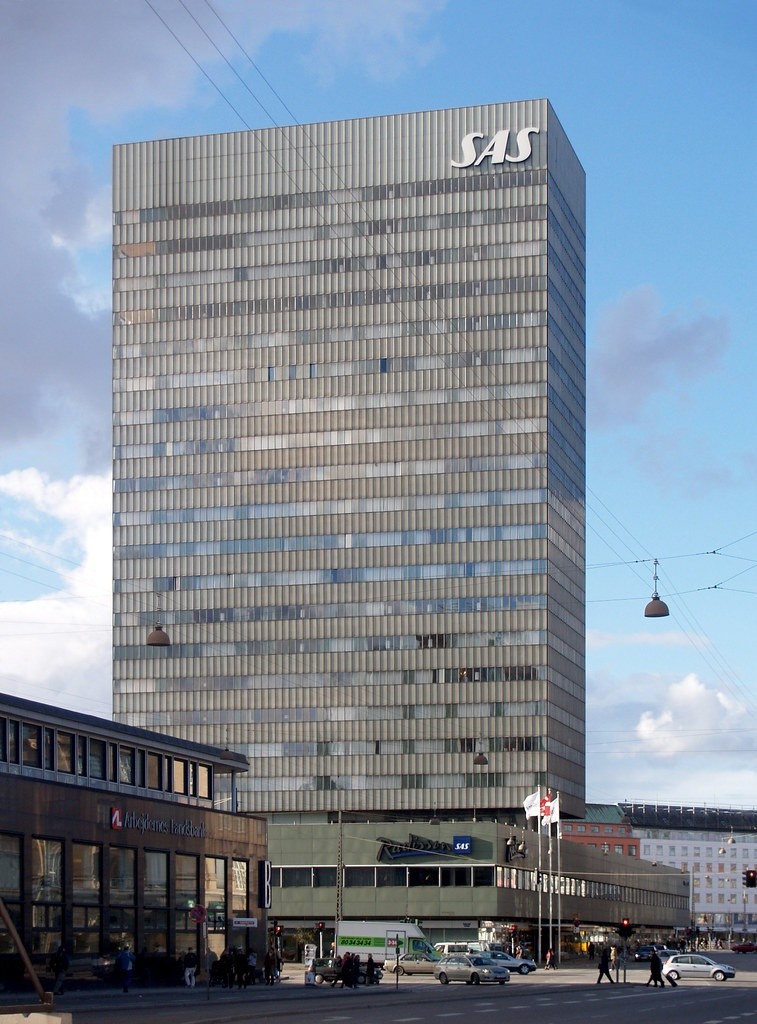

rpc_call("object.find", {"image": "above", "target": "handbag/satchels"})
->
[598,964,600,969]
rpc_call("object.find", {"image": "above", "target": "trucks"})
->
[333,920,437,971]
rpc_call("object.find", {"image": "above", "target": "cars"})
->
[634,945,658,962]
[383,952,441,976]
[656,949,680,965]
[660,953,736,982]
[433,954,511,985]
[307,957,384,985]
[731,942,757,954]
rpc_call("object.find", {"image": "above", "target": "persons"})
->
[118,947,136,992]
[597,945,622,983]
[178,947,197,988]
[516,946,523,960]
[588,942,595,960]
[331,952,361,988]
[545,949,558,970]
[205,947,284,990]
[646,951,665,988]
[714,937,723,949]
[650,938,708,954]
[476,958,482,965]
[49,947,69,994]
[366,954,379,986]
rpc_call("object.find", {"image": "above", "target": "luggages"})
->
[661,971,677,987]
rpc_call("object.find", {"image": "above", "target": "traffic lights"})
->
[318,922,325,931]
[275,925,284,936]
[622,918,629,936]
[746,871,757,888]
[742,871,747,887]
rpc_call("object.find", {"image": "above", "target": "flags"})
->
[542,798,558,826]
[523,792,540,819]
[540,794,550,816]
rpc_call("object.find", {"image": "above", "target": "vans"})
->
[426,942,469,957]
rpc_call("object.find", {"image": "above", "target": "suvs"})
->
[470,950,537,975]
